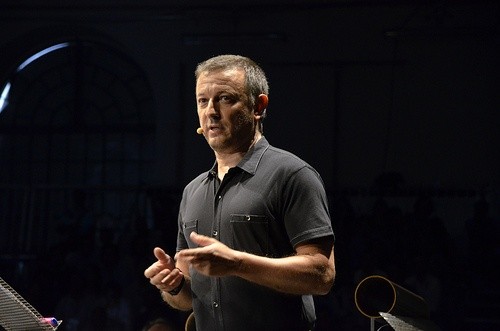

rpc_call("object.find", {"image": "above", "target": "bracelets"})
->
[168,277,185,296]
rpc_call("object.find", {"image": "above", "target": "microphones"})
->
[197,128,203,134]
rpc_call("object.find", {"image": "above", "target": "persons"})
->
[144,53,337,331]
